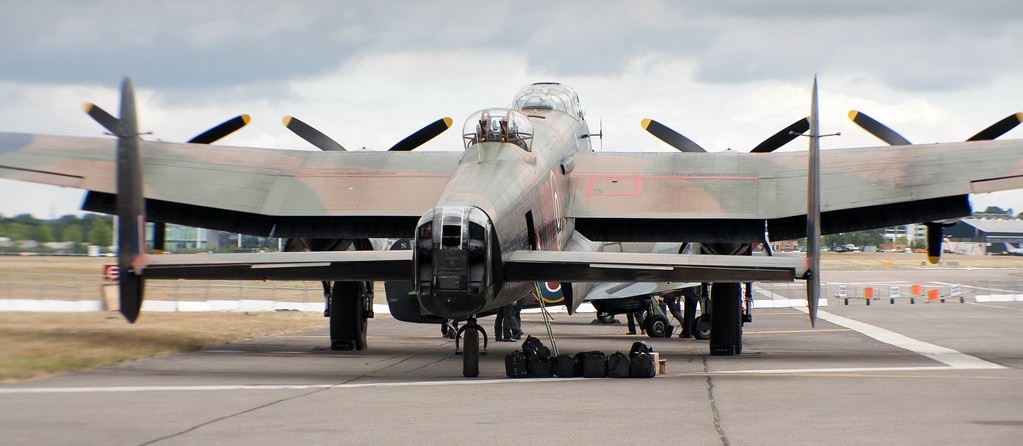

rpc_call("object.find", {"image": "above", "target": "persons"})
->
[441,322,462,338]
[656,295,684,338]
[626,312,646,335]
[494,309,524,342]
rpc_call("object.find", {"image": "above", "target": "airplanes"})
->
[0,73,1023,380]
[1003,241,1023,256]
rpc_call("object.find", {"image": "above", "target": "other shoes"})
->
[642,329,646,334]
[512,330,524,339]
[443,334,449,337]
[504,337,516,342]
[496,338,504,341]
[450,335,461,339]
[626,331,636,335]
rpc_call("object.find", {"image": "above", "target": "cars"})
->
[819,243,878,253]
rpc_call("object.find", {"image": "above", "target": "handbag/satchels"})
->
[505,335,656,378]
